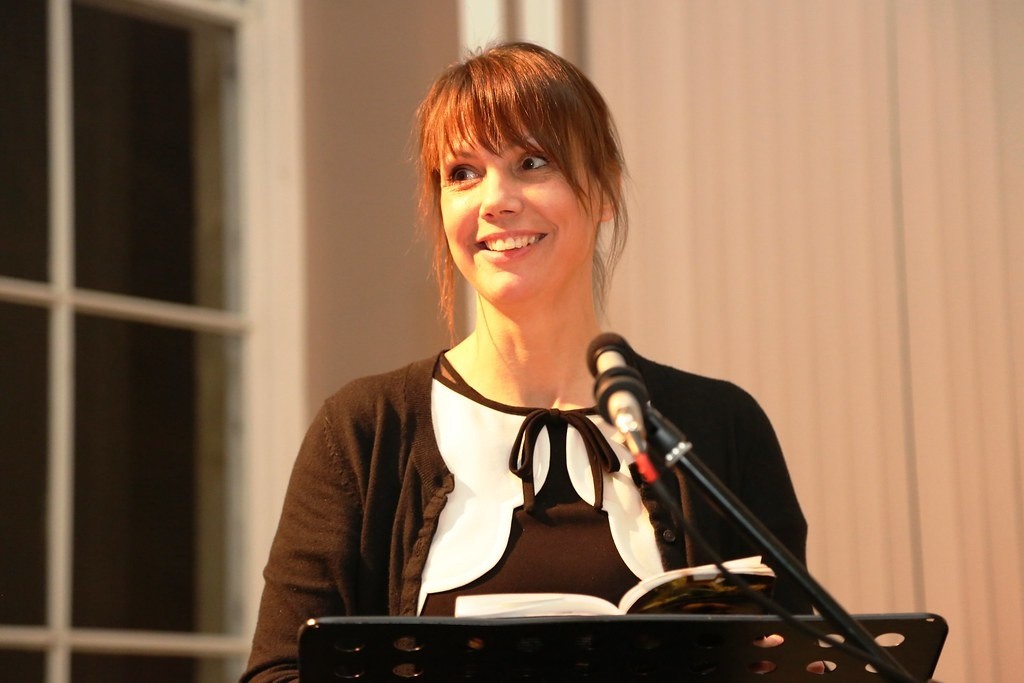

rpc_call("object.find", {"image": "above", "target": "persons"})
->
[238,42,836,683]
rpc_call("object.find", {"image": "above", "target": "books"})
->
[455,553,777,618]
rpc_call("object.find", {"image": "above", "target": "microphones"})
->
[588,332,646,456]
